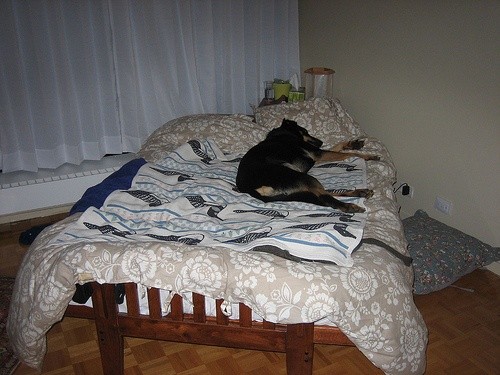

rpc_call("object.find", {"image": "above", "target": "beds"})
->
[10,96,428,375]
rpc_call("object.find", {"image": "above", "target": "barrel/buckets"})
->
[304,67,336,98]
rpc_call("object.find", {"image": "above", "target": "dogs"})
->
[232,117,381,214]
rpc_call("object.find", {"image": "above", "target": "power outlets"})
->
[433,195,451,214]
[402,185,414,199]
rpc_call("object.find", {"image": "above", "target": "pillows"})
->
[252,97,364,159]
[397,208,500,296]
[140,114,271,155]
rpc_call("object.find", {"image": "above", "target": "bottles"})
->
[264,81,274,99]
